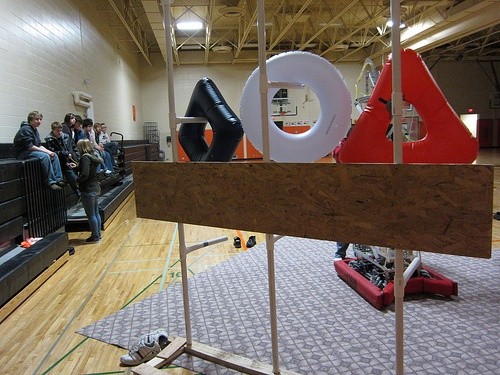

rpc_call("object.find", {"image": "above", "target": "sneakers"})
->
[132,328,168,351]
[120,340,161,365]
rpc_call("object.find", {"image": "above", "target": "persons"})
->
[332,123,393,262]
[76,138,102,244]
[12,111,123,191]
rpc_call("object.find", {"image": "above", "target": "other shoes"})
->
[493,212,500,220]
[105,170,111,174]
[84,238,99,243]
[234,237,241,248]
[247,236,256,247]
[51,182,67,191]
